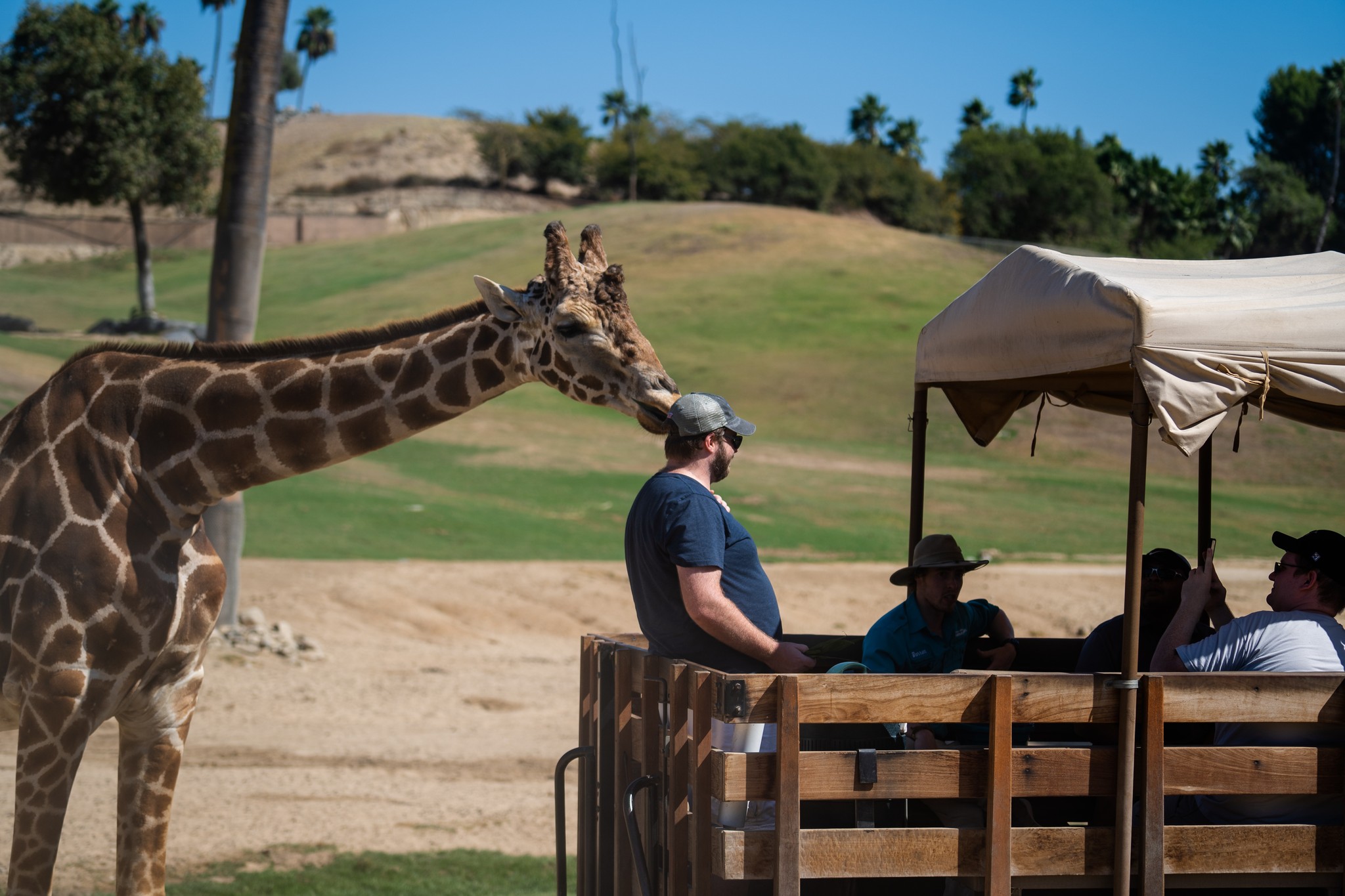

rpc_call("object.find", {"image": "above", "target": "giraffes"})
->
[1,221,691,895]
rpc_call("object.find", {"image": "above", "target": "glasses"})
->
[1274,562,1314,574]
[1142,566,1187,580]
[702,431,743,449]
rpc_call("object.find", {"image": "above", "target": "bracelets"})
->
[910,725,934,741]
[1003,637,1020,654]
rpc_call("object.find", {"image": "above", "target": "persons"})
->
[623,392,818,830]
[863,534,1029,750]
[1147,528,1345,674]
[1077,546,1217,673]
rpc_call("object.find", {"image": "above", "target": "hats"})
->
[1272,529,1345,585]
[1142,548,1192,572]
[667,391,756,437]
[889,534,989,586]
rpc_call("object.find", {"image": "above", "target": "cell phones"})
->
[1201,538,1217,570]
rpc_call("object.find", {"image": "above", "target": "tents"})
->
[909,244,1345,604]
